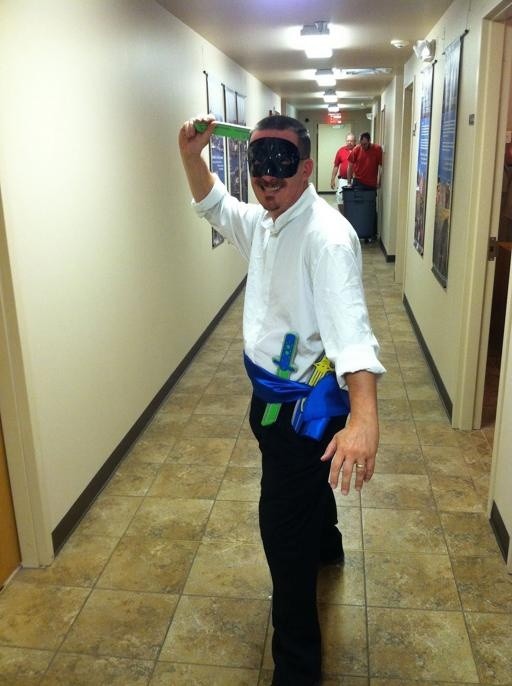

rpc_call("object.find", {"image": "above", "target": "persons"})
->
[330,133,357,215]
[347,131,383,193]
[180,113,388,686]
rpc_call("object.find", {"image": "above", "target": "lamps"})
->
[323,89,337,103]
[315,68,337,87]
[328,104,339,114]
[299,22,333,59]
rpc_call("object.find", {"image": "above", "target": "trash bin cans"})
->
[342,186,378,244]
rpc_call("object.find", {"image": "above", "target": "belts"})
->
[338,175,353,179]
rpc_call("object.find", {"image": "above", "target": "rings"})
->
[355,464,365,468]
